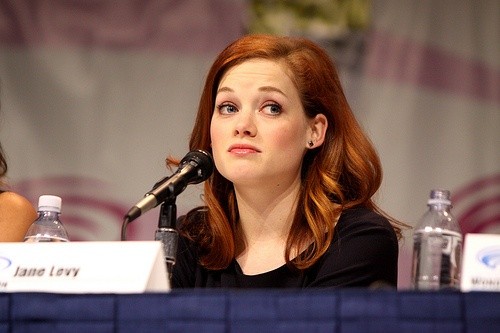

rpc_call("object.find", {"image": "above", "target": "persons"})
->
[0,143,38,242]
[173,35,399,293]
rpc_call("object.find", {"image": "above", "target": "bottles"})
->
[410,189,463,292]
[22,195,70,243]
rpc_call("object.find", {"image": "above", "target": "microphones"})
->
[125,149,214,222]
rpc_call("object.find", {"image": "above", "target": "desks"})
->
[0,293,500,333]
